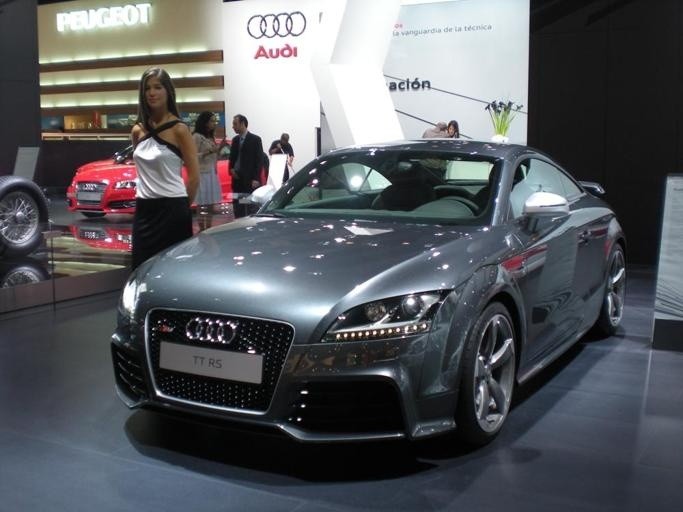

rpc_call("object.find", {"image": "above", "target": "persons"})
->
[268,133,294,188]
[225,114,264,222]
[188,110,227,232]
[446,119,460,141]
[131,67,202,271]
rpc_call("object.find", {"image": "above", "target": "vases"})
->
[491,134,510,144]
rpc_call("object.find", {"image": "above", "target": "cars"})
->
[109,138,630,449]
[0,171,52,260]
[74,141,136,176]
[64,139,268,218]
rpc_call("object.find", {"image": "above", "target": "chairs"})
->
[371,157,527,221]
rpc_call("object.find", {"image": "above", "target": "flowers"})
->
[483,98,524,132]
